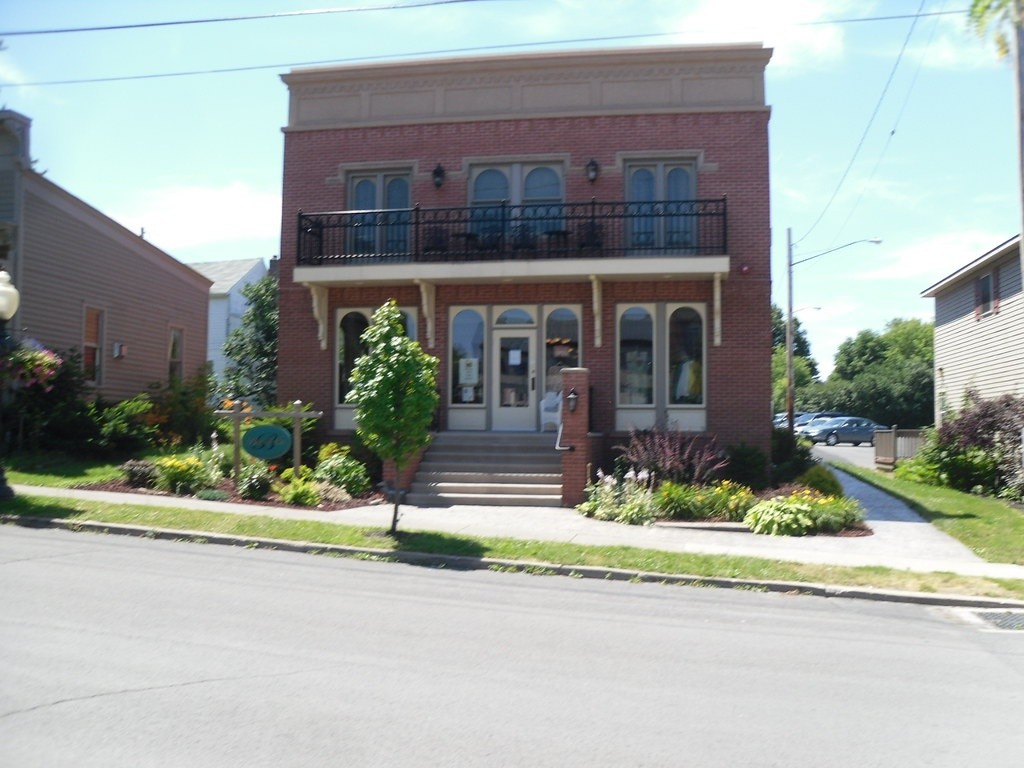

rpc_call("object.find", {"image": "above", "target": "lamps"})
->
[431,162,449,189]
[586,157,599,183]
[564,388,579,411]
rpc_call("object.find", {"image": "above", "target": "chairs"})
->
[577,221,605,258]
[421,226,449,263]
[509,222,540,259]
[476,224,506,259]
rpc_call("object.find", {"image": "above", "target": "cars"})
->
[774,412,888,446]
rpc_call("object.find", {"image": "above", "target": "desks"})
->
[451,233,480,261]
[544,230,574,258]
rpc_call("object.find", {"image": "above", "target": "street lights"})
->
[787,237,883,451]
